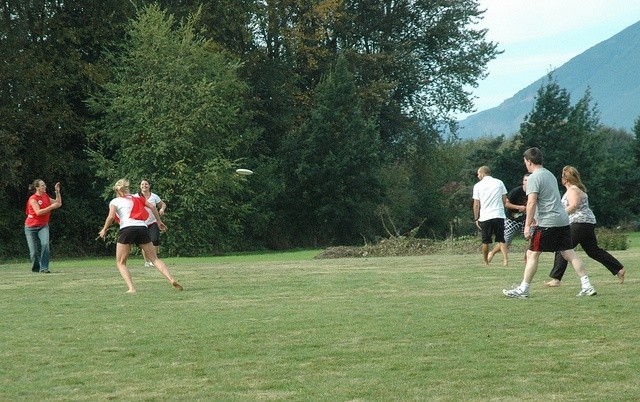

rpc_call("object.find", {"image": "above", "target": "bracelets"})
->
[474,218,478,222]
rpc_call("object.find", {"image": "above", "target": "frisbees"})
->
[236,168,254,176]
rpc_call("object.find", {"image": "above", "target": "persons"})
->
[133,180,167,267]
[503,147,597,299]
[543,165,625,287]
[472,165,508,266]
[98,178,183,293]
[488,173,531,269]
[24,179,62,273]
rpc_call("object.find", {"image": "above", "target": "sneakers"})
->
[502,286,528,298]
[575,285,597,297]
